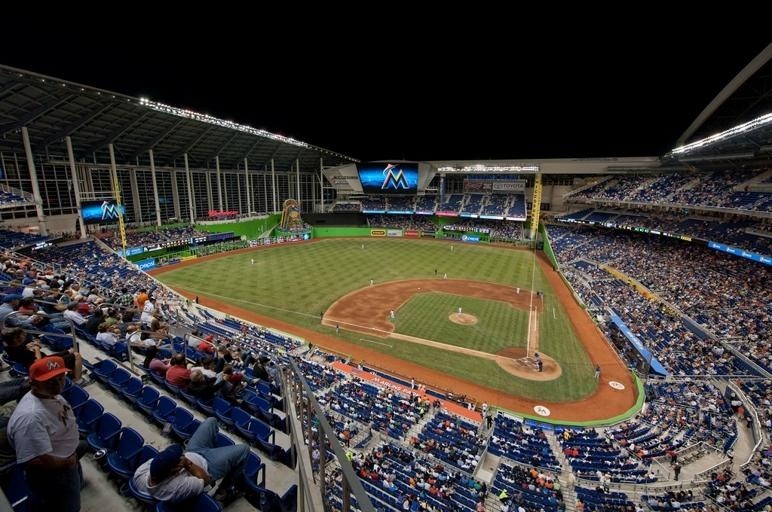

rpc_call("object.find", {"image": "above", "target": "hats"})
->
[231,373,243,383]
[29,355,72,383]
[2,293,24,303]
[139,289,147,292]
[259,356,270,363]
[206,336,213,341]
[150,297,157,301]
[150,444,183,482]
[223,367,236,373]
[120,288,129,291]
[49,279,61,288]
[201,357,211,369]
[217,345,226,351]
[153,313,163,318]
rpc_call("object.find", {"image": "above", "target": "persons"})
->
[0,163,772,512]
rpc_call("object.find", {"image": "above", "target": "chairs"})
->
[1,302,290,511]
